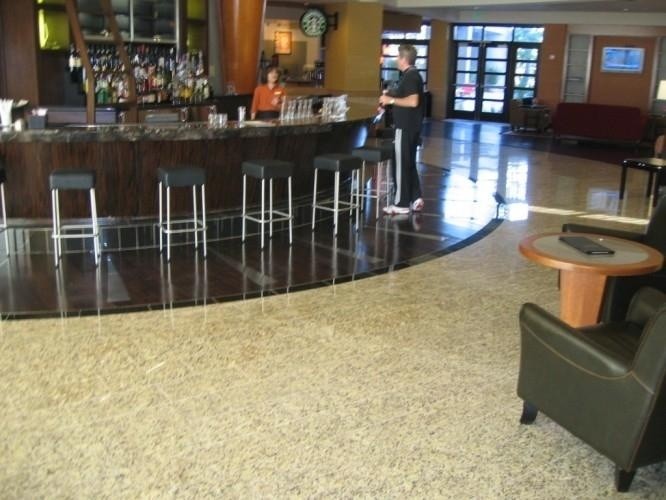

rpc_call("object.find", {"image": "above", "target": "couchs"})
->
[552,103,650,153]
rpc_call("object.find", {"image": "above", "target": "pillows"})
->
[532,97,545,105]
[523,97,533,103]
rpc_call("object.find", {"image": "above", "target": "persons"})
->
[248,65,286,121]
[377,44,424,214]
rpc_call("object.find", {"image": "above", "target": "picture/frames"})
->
[274,30,293,56]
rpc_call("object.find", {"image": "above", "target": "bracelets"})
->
[389,97,395,105]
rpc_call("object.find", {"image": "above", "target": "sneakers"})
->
[383,204,410,214]
[413,198,424,211]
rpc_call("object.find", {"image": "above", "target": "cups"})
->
[237,106,245,128]
[207,113,228,129]
[278,96,349,120]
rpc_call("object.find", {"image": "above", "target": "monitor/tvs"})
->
[600,46,645,73]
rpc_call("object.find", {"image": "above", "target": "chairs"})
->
[509,99,551,135]
[517,284,665,492]
[557,194,666,325]
[619,135,666,206]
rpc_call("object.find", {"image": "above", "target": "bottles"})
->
[66,41,209,104]
[379,90,389,109]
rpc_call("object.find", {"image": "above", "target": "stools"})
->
[157,163,208,262]
[0,168,9,229]
[49,168,102,268]
[241,158,294,249]
[311,126,395,238]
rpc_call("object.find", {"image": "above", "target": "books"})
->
[560,236,615,255]
